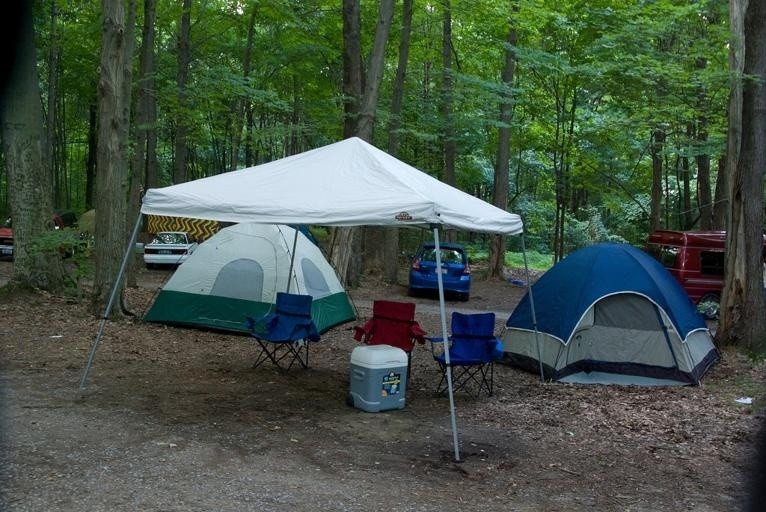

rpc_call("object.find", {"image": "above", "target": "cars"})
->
[142,231,195,270]
[405,240,471,302]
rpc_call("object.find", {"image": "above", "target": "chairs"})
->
[240,293,320,376]
[424,311,496,401]
[352,299,428,391]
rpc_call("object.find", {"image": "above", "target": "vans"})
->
[645,228,766,318]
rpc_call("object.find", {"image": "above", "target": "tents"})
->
[498,243,722,386]
[141,222,357,335]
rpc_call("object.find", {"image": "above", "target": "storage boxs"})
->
[350,345,409,412]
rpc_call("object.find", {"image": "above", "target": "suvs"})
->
[0,207,80,261]
